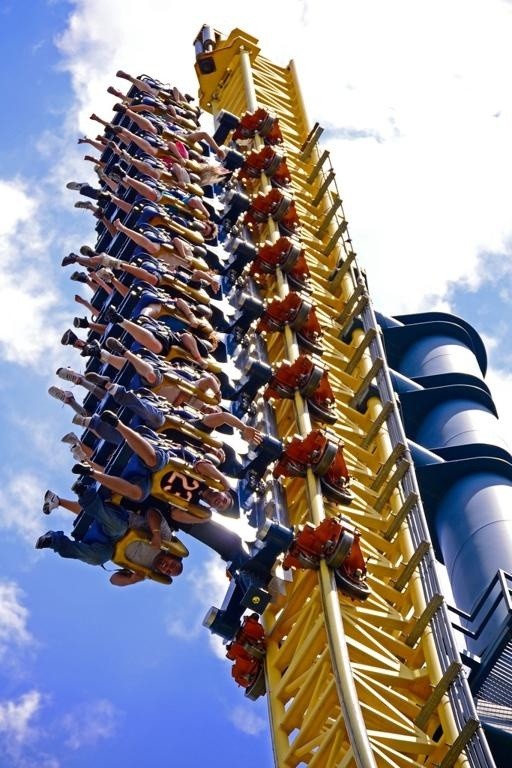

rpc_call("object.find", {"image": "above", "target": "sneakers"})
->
[35,67,133,550]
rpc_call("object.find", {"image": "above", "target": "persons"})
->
[37,68,263,587]
